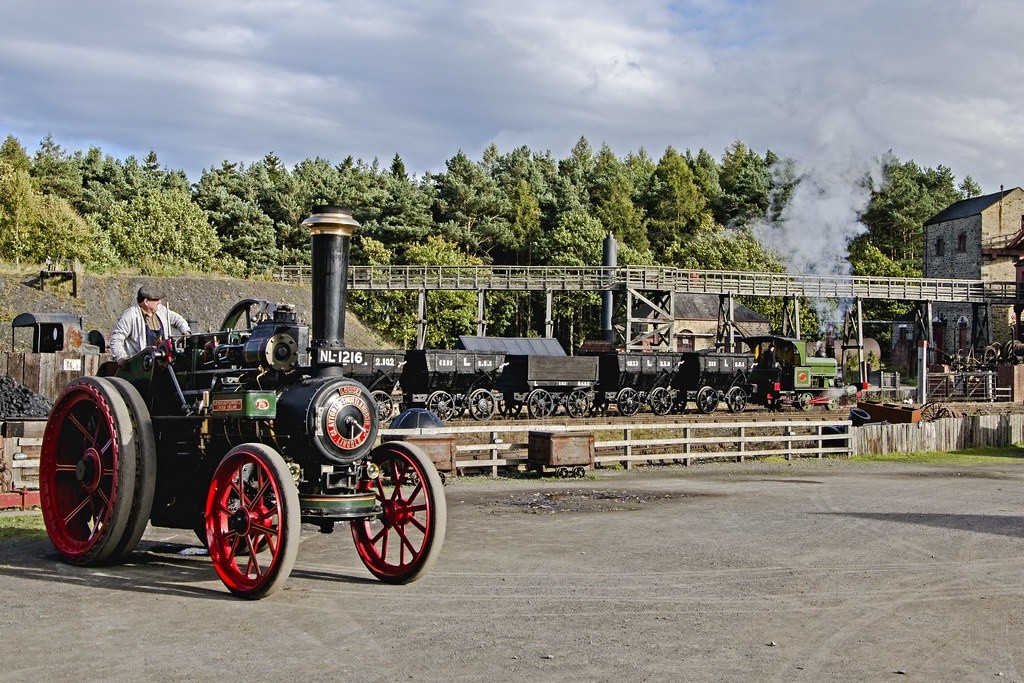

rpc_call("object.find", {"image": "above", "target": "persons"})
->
[110,286,192,371]
[763,344,784,371]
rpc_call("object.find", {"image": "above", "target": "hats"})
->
[138,286,165,300]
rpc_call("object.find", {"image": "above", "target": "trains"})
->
[347,320,837,422]
